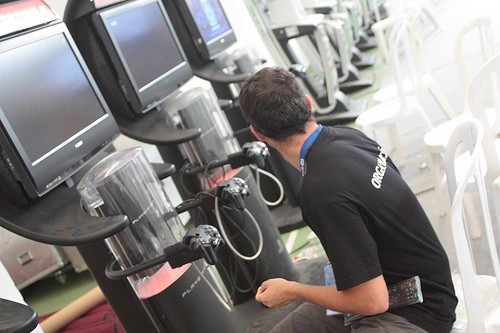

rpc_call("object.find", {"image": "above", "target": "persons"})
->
[238,68,458,333]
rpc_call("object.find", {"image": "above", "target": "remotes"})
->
[344,276,423,323]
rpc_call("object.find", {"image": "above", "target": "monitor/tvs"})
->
[68,0,194,127]
[0,17,121,208]
[162,0,238,67]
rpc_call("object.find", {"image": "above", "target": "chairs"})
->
[353,0,500,333]
